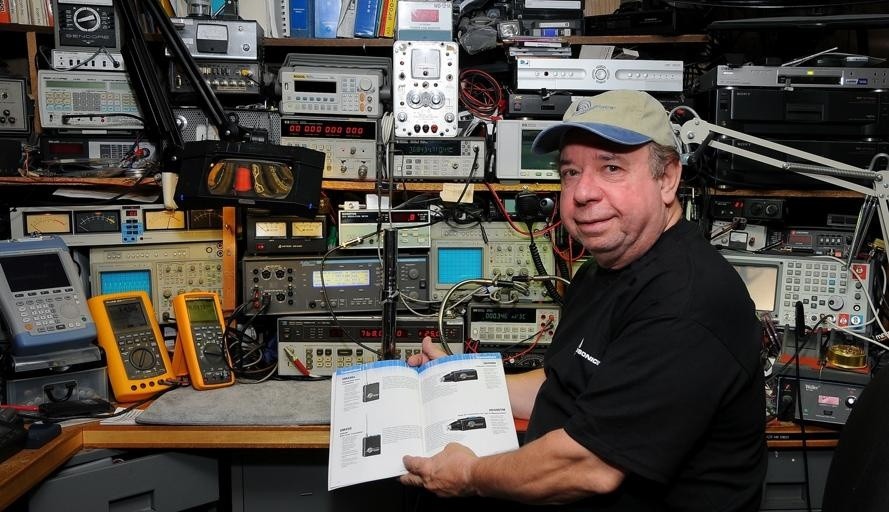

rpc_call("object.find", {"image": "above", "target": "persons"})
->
[392,88,769,511]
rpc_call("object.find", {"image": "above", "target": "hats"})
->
[531,89,680,155]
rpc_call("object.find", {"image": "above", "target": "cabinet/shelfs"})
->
[0,21,888,508]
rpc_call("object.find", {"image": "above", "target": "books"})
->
[0,0,56,27]
[209,1,398,40]
[324,352,521,492]
